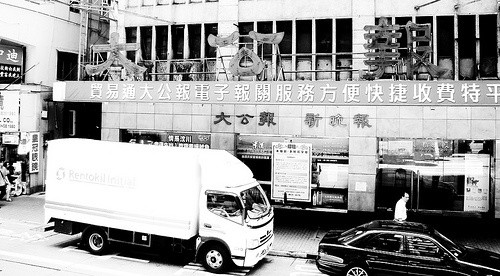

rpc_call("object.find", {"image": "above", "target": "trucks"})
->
[43,138,276,274]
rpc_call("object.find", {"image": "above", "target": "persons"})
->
[207,194,226,217]
[0,159,11,208]
[3,162,16,202]
[394,192,410,221]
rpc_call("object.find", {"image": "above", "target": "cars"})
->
[315,219,500,276]
